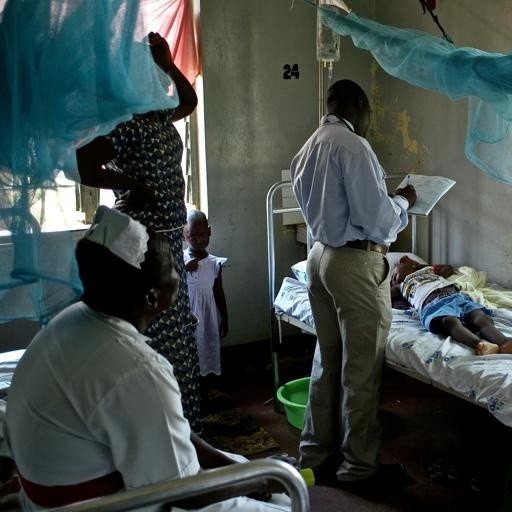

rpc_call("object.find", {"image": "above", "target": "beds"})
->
[265,181,512,430]
[0,315,311,512]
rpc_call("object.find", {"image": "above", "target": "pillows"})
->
[291,249,429,283]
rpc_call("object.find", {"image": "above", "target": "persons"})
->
[289,80,416,485]
[390,256,512,356]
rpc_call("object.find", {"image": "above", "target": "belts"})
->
[346,240,388,254]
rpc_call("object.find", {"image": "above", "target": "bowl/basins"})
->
[275,375,309,430]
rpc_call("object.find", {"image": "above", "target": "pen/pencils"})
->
[407,176,410,186]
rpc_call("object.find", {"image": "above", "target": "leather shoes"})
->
[300,457,407,498]
